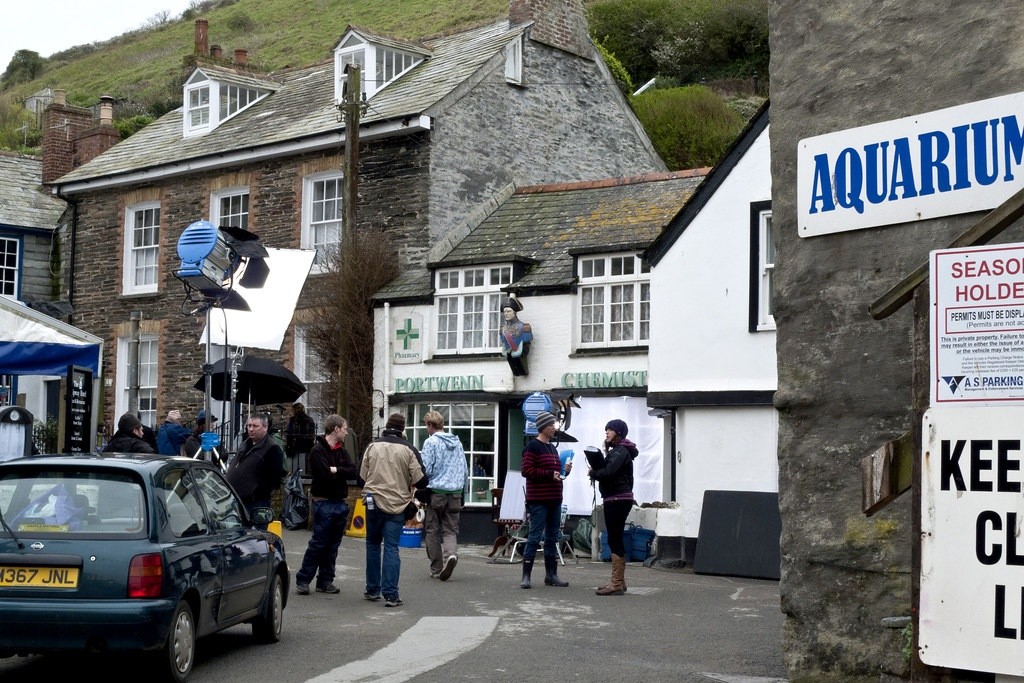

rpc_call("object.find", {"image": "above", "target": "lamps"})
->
[176,221,270,311]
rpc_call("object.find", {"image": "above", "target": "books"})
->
[560,450,576,480]
[584,447,605,470]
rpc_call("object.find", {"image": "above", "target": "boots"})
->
[596,554,627,595]
[521,557,534,587]
[544,560,569,587]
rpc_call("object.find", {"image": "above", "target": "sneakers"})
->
[316,584,340,594]
[385,595,402,607]
[296,582,310,593]
[364,591,382,599]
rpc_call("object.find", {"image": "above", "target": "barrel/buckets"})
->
[598,525,657,563]
[398,528,422,548]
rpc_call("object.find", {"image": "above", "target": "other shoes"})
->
[429,570,440,578]
[440,555,457,581]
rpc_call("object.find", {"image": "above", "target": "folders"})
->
[559,449,575,478]
[584,446,605,471]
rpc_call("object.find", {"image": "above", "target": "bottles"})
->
[366,493,374,510]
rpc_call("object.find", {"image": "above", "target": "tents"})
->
[0,295,105,456]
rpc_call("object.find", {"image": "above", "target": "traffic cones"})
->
[345,499,366,539]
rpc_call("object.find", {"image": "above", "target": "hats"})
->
[197,410,219,425]
[169,410,182,419]
[605,420,629,439]
[387,413,405,431]
[118,414,141,432]
[534,411,556,430]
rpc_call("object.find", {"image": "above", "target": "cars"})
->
[0,454,292,682]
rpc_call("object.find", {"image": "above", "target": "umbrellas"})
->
[193,354,306,418]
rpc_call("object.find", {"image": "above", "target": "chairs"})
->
[489,488,517,557]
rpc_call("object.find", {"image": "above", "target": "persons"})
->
[185,409,228,468]
[297,414,360,596]
[419,410,469,581]
[286,402,316,457]
[223,413,289,510]
[137,409,157,450]
[155,409,193,455]
[588,418,638,595]
[520,412,573,588]
[102,412,155,456]
[496,296,534,375]
[359,412,429,607]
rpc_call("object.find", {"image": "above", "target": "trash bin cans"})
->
[399,529,423,548]
